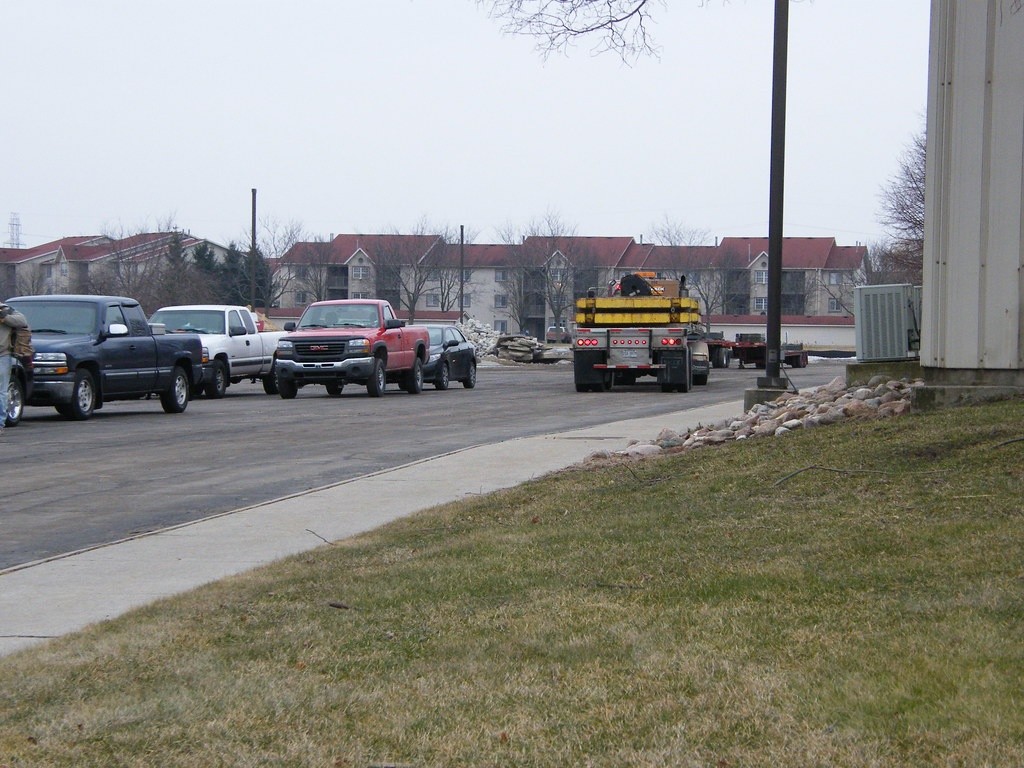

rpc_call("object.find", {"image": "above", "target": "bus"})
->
[571,272,712,393]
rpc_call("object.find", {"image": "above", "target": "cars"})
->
[419,324,479,391]
[546,326,572,344]
[3,354,34,427]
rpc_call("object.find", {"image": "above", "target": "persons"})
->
[325,312,338,325]
[369,313,377,325]
[0,302,35,434]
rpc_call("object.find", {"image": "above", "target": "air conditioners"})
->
[855,283,920,363]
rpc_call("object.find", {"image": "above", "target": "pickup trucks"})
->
[275,300,432,400]
[147,304,290,399]
[4,294,204,420]
